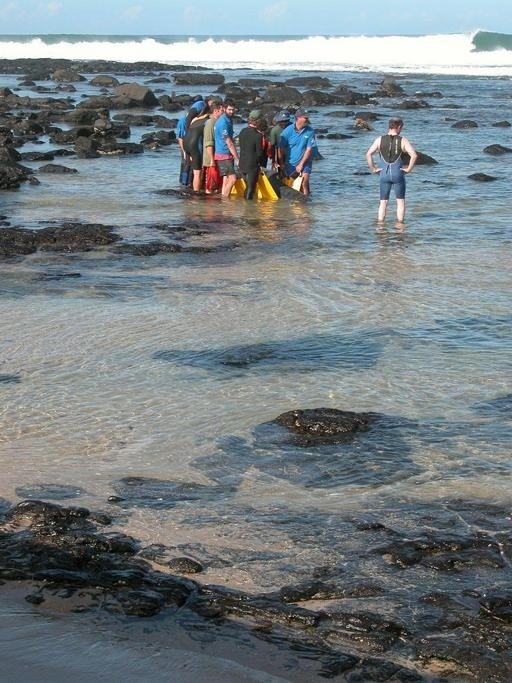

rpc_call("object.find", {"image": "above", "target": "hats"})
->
[249,110,264,121]
[276,110,292,122]
[294,108,311,118]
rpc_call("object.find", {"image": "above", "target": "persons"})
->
[365,116,418,222]
[175,96,321,201]
